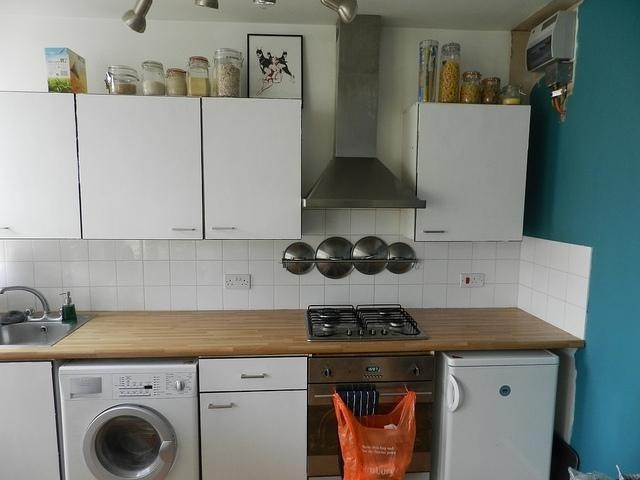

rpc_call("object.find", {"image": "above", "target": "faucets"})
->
[0,285,50,320]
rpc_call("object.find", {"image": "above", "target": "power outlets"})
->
[224,274,251,289]
[460,273,485,288]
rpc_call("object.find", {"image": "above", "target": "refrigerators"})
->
[436,350,560,480]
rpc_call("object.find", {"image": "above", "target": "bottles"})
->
[106,65,137,95]
[439,43,460,102]
[419,40,439,102]
[502,84,522,104]
[213,48,243,97]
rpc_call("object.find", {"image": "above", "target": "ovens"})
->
[307,355,435,480]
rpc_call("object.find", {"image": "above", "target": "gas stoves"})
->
[305,304,425,340]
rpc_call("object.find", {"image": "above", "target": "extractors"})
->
[301,16,427,209]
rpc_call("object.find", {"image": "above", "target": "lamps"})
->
[122,0,358,33]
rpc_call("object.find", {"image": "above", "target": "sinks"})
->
[0,321,75,347]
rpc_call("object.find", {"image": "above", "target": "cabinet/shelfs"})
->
[75,92,302,241]
[0,91,82,240]
[402,101,532,243]
[198,357,308,479]
[0,361,61,479]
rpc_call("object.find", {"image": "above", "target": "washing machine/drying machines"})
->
[58,361,200,480]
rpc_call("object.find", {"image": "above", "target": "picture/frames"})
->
[247,34,303,99]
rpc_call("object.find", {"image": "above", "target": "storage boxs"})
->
[44,48,87,93]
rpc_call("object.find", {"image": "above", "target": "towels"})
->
[335,390,380,480]
